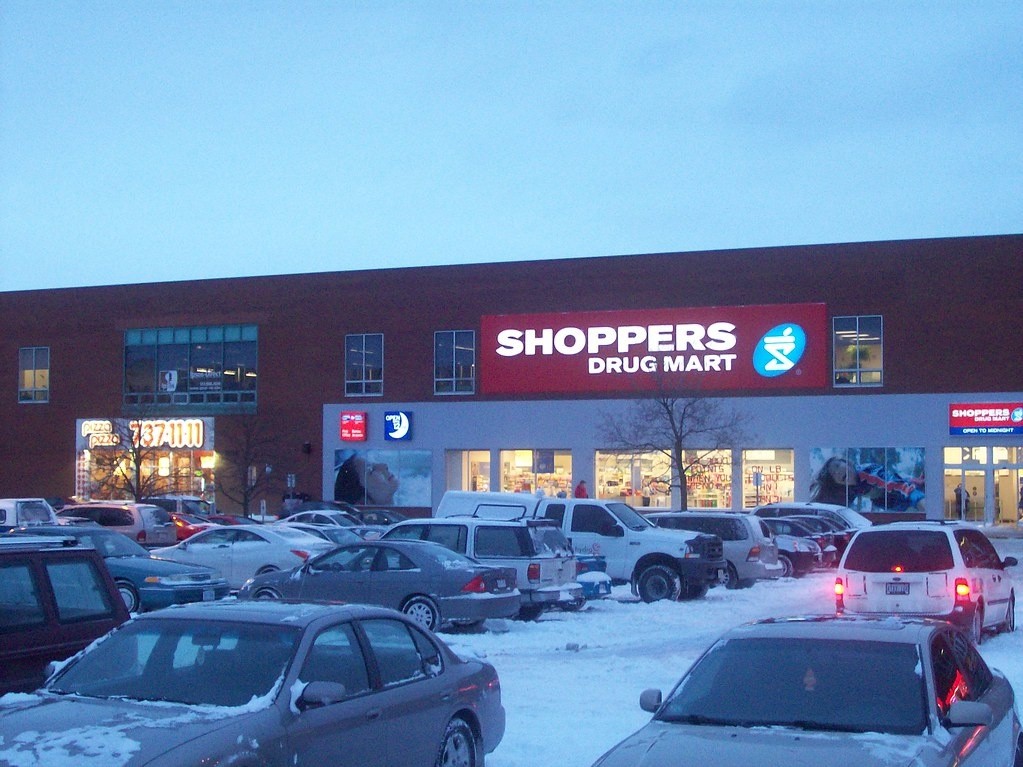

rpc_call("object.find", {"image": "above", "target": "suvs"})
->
[834,518,1018,645]
[340,514,583,623]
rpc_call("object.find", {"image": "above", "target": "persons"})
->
[640,482,650,507]
[954,484,970,520]
[808,457,927,512]
[574,480,588,498]
[334,454,400,507]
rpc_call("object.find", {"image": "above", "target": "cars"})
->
[204,515,262,527]
[557,552,614,613]
[170,511,216,542]
[236,539,520,635]
[280,500,358,519]
[0,523,233,615]
[0,599,506,767]
[758,514,855,579]
[591,615,1023,767]
[153,510,407,592]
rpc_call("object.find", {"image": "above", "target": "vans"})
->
[643,512,783,590]
[747,500,872,531]
[0,498,62,529]
[53,502,178,549]
[0,549,138,701]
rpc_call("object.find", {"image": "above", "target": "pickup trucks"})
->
[433,490,726,604]
